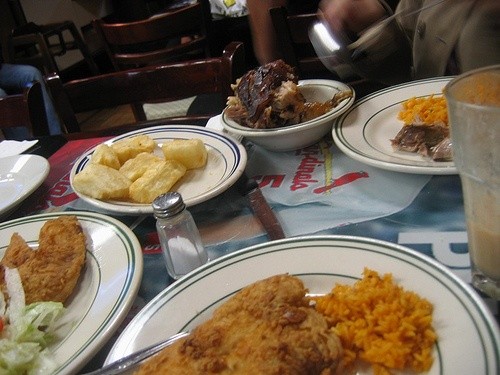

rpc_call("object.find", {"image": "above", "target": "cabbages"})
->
[0,267,78,375]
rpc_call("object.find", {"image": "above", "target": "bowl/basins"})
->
[220,79,356,152]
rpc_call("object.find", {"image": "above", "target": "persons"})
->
[0,45,63,135]
[100,0,500,94]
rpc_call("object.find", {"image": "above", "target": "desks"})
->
[147,0,252,59]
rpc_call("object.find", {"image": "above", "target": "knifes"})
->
[236,171,285,241]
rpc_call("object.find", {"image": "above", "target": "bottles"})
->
[151,191,210,280]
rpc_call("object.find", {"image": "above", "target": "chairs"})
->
[4,0,107,99]
[54,40,247,133]
[0,80,51,138]
[268,5,372,101]
[89,0,234,122]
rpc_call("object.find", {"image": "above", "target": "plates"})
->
[69,124,247,214]
[101,235,500,375]
[0,211,144,375]
[0,154,51,214]
[331,76,458,175]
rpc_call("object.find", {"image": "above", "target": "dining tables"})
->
[0,125,500,375]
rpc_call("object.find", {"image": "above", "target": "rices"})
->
[313,267,436,375]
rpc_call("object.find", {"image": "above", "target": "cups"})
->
[444,64,500,298]
